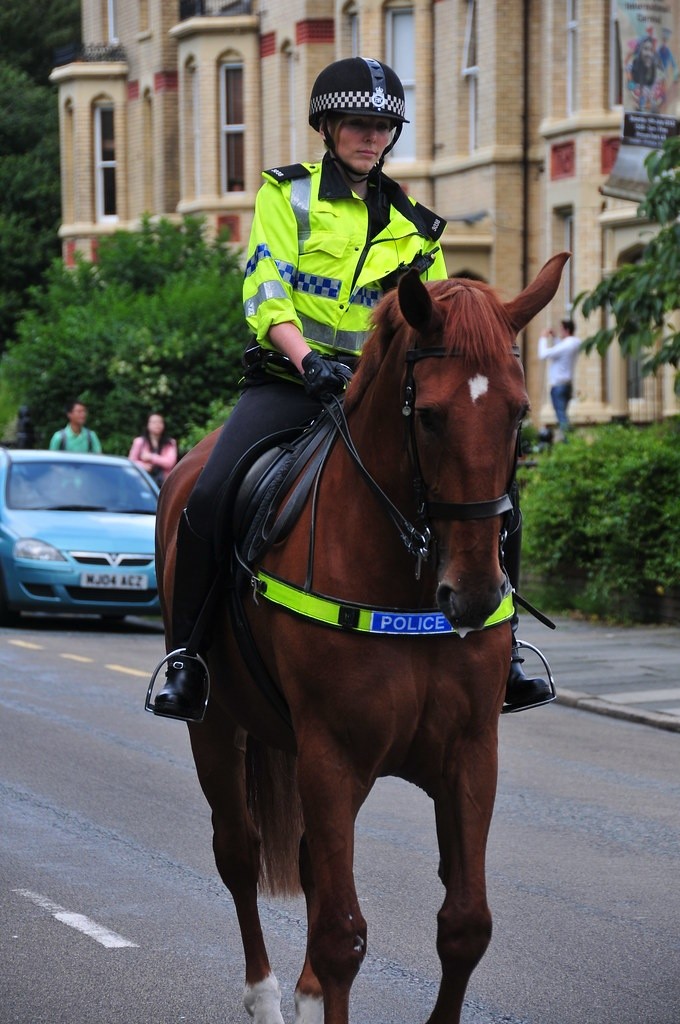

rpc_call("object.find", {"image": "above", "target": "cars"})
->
[0,447,164,627]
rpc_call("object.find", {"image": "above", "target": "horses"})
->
[146,244,580,1024]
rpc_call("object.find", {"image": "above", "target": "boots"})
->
[498,507,551,711]
[154,508,233,721]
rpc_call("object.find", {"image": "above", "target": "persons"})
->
[49,400,102,456]
[154,57,554,720]
[538,320,581,444]
[128,411,178,489]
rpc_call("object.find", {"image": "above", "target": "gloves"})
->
[301,349,355,398]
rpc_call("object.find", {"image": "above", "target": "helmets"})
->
[307,55,411,132]
